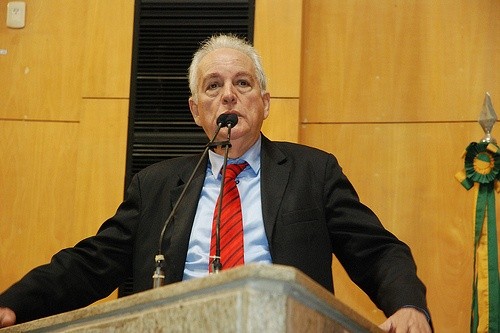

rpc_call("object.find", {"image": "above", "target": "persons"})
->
[0,32,433,333]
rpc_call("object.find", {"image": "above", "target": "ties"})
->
[208,161,250,274]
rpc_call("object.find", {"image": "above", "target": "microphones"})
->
[212,113,238,273]
[153,113,227,289]
